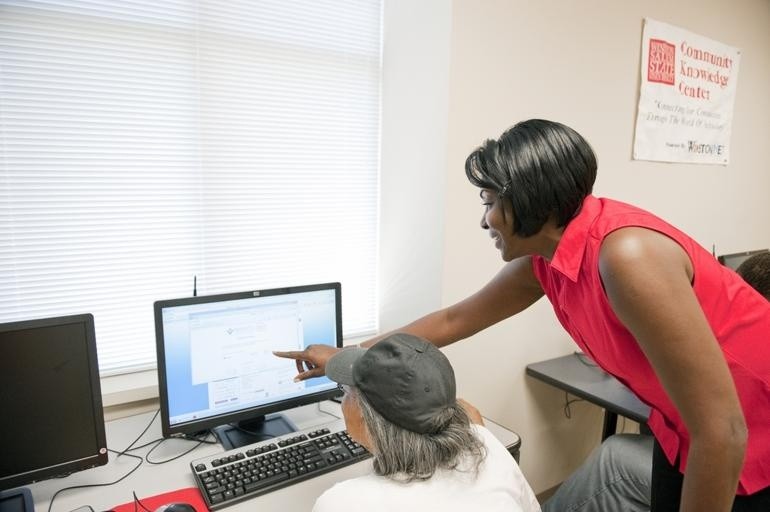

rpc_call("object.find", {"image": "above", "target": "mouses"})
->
[154,502,196,512]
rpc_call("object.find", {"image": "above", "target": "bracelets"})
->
[355,342,361,348]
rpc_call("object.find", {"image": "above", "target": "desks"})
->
[526,350,653,442]
[1,378,521,510]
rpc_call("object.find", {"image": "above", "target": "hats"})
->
[324,333,456,434]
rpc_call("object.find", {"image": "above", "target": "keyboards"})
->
[191,419,374,512]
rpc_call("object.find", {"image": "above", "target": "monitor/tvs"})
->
[154,281,344,452]
[0,312,108,512]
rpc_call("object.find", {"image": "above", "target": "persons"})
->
[271,119,770,512]
[311,333,542,512]
[539,251,770,512]
